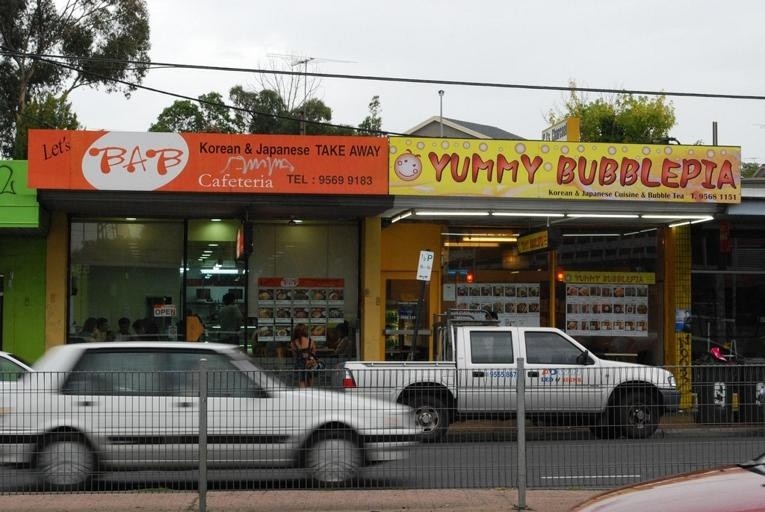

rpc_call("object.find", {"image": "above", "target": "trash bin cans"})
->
[692,357,765,423]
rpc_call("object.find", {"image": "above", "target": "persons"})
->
[74,307,207,342]
[289,323,318,388]
[485,311,500,326]
[330,321,354,387]
[217,291,245,346]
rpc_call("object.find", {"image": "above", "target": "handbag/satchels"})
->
[307,355,325,375]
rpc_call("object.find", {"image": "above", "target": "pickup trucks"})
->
[333,325,681,438]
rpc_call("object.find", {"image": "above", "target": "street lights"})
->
[439,90,445,137]
[296,56,314,132]
